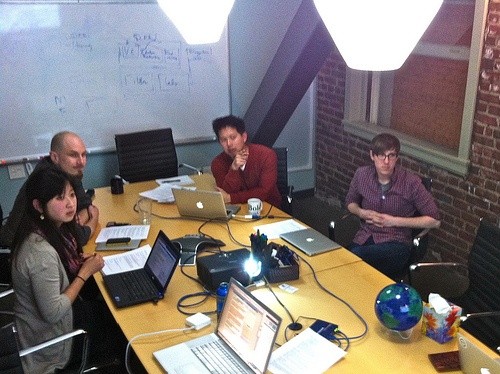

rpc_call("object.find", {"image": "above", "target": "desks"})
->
[82,175,500,374]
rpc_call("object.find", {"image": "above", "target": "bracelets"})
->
[76,275,86,284]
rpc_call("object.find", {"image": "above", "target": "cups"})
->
[109,178,124,194]
[247,198,263,219]
[136,197,152,225]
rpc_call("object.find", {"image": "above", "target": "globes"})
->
[373,279,424,340]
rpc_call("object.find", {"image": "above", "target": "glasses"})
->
[371,152,397,160]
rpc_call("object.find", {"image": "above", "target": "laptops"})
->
[153,276,283,374]
[280,228,342,255]
[172,187,242,221]
[104,230,182,309]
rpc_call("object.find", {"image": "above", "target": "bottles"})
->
[216,282,229,318]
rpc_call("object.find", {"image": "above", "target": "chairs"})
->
[408,219,500,356]
[0,204,118,374]
[113,129,202,185]
[330,213,430,273]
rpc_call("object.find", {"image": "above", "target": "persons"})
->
[345,133,442,278]
[211,115,283,210]
[0,132,148,374]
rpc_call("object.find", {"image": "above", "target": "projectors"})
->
[196,248,263,291]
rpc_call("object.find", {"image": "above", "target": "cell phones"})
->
[106,236,132,246]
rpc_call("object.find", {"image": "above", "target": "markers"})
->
[23,156,42,161]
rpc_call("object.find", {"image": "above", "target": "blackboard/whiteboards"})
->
[0,0,233,167]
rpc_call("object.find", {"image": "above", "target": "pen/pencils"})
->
[162,180,181,183]
[251,229,268,241]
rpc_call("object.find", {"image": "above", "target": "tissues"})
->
[421,293,462,344]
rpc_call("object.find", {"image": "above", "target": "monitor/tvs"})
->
[457,330,500,374]
